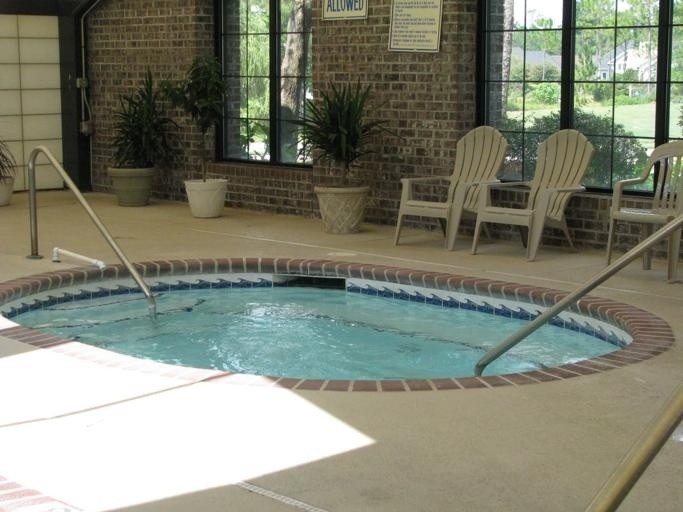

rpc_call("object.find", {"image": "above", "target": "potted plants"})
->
[0,139,19,207]
[285,73,406,236]
[102,53,235,219]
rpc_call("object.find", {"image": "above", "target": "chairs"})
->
[471,129,594,263]
[394,125,507,251]
[606,140,683,283]
